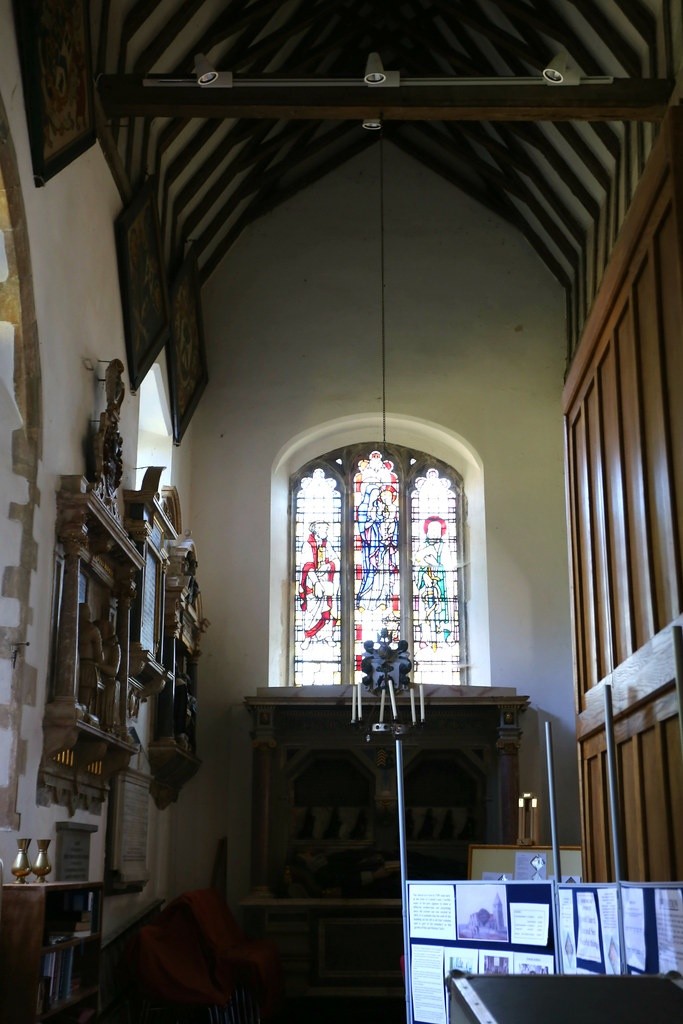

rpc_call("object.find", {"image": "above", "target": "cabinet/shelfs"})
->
[0,880,106,1024]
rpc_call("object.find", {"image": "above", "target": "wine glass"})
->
[11,838,52,885]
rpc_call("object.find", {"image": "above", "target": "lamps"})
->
[193,50,220,87]
[544,53,573,83]
[360,118,382,131]
[363,51,387,85]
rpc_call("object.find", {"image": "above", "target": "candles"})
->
[351,679,428,725]
